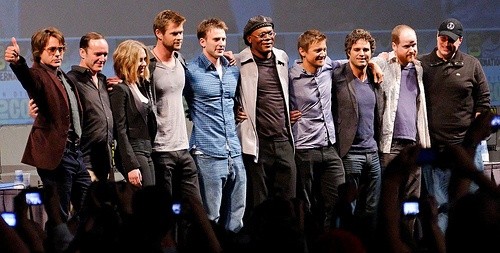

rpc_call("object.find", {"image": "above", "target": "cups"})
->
[22,173,30,189]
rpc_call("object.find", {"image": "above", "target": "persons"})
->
[0,112,500,253]
[4,10,497,233]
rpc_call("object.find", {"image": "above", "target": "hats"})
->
[243,15,275,34]
[437,18,463,41]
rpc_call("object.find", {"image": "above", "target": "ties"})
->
[58,71,82,140]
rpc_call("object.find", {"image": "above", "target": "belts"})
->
[66,140,80,150]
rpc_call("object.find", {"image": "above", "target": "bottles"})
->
[14,170,25,189]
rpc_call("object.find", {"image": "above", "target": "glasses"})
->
[249,32,277,40]
[45,45,67,54]
